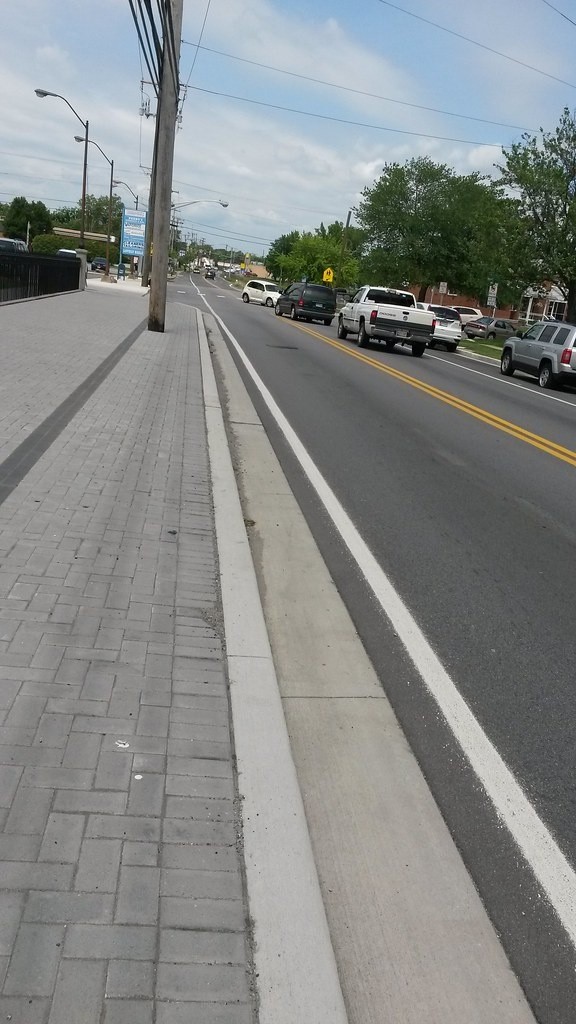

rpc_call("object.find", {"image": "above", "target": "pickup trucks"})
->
[335,283,437,357]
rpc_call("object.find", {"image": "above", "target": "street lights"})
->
[74,135,114,276]
[113,179,139,274]
[34,88,90,249]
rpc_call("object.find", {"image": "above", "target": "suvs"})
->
[274,282,337,326]
[500,319,576,389]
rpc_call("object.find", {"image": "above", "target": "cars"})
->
[409,301,463,353]
[193,264,235,279]
[444,304,484,327]
[0,237,30,253]
[463,316,521,341]
[91,256,109,273]
[57,248,77,258]
[241,279,284,308]
